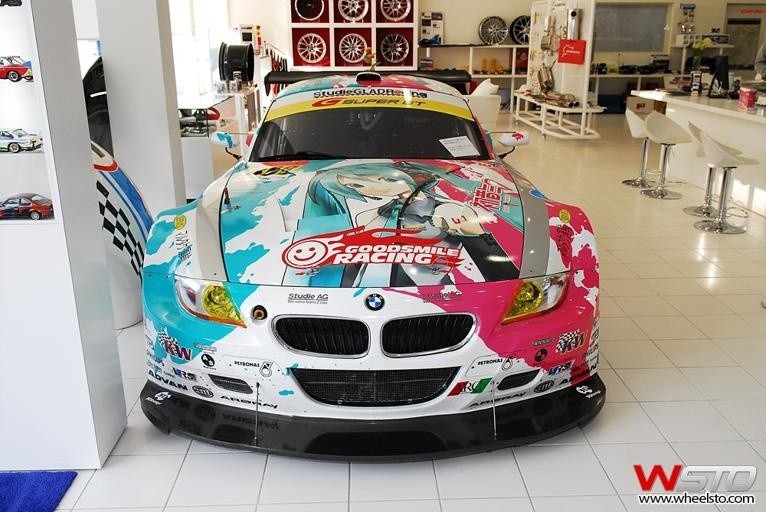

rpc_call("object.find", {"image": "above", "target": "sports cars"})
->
[139,71,607,463]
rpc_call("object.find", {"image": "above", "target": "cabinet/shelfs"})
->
[178,80,261,199]
[287,0,735,140]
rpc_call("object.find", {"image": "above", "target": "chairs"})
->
[622,108,750,234]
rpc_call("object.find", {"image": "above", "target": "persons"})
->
[754,39,766,81]
[307,160,520,287]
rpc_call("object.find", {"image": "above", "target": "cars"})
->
[0,193,53,220]
[0,128,43,153]
[0,55,33,82]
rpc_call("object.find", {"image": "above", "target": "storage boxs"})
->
[627,97,666,121]
